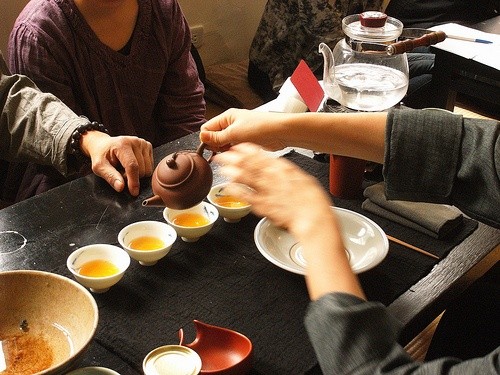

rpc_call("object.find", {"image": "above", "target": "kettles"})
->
[318,11,446,111]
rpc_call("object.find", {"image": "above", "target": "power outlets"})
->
[190,25,205,49]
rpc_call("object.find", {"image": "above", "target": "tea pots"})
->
[142,142,217,210]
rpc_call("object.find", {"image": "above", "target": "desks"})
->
[429,15,500,123]
[0,131,500,375]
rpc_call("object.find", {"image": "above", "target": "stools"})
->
[204,57,277,113]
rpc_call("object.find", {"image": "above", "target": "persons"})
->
[9,0,207,204]
[0,51,155,196]
[200,0,500,375]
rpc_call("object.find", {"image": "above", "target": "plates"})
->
[254,206,389,275]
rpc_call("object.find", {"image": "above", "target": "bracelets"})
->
[71,121,109,161]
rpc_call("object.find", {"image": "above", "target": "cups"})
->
[0,231,26,271]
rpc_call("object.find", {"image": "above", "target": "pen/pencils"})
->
[445,34,493,44]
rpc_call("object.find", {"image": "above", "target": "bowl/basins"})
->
[179,320,253,375]
[66,244,131,294]
[163,201,219,242]
[118,221,177,266]
[0,270,99,374]
[207,184,256,223]
